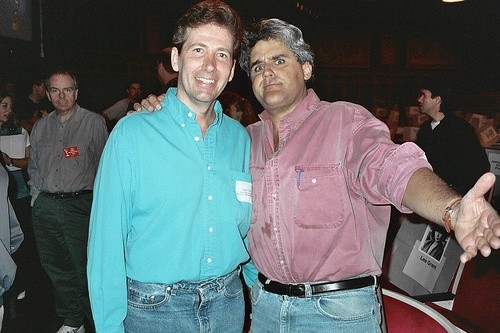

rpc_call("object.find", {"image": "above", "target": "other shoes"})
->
[17,290,25,299]
[56,324,86,333]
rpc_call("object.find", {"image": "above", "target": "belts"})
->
[258,272,378,297]
[41,189,92,199]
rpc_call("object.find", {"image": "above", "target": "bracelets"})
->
[442,199,463,233]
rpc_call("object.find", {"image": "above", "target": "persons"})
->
[86,0,261,332]
[402,81,491,240]
[0,161,24,333]
[101,79,144,130]
[0,94,52,308]
[128,46,179,114]
[422,223,447,261]
[216,78,259,128]
[21,76,56,135]
[27,69,109,333]
[126,17,499,333]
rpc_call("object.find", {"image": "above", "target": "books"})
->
[0,134,26,171]
